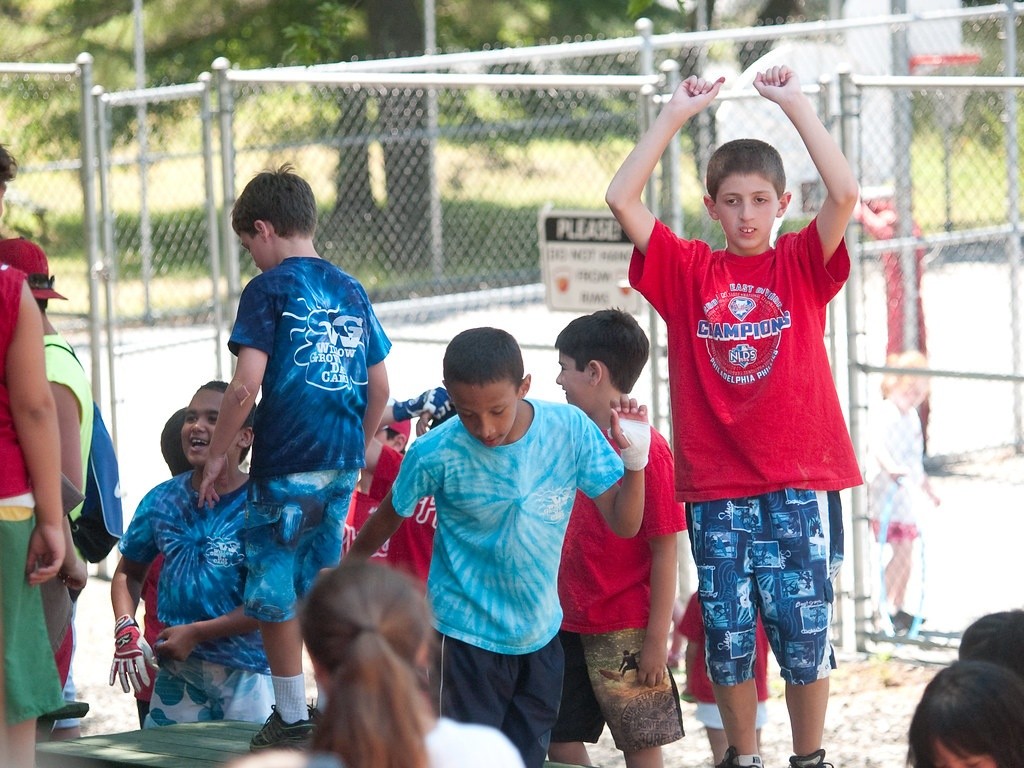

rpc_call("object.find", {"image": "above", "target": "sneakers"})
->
[249,705,318,752]
[307,699,322,721]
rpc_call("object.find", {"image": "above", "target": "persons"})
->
[547,309,685,768]
[198,162,392,750]
[605,64,865,768]
[110,380,314,730]
[317,326,650,768]
[0,236,94,741]
[666,605,683,667]
[0,146,68,768]
[677,590,770,768]
[906,660,1024,768]
[134,407,191,730]
[866,349,939,631]
[958,610,1024,665]
[237,564,524,768]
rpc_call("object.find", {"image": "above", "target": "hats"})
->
[377,397,411,437]
[0,236,68,300]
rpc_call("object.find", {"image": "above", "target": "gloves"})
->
[109,614,159,692]
[394,387,452,422]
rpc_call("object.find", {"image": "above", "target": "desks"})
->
[35,721,592,768]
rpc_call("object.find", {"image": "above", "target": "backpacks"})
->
[44,337,124,562]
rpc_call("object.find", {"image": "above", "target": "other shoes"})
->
[732,754,764,768]
[872,612,922,627]
[788,749,835,768]
